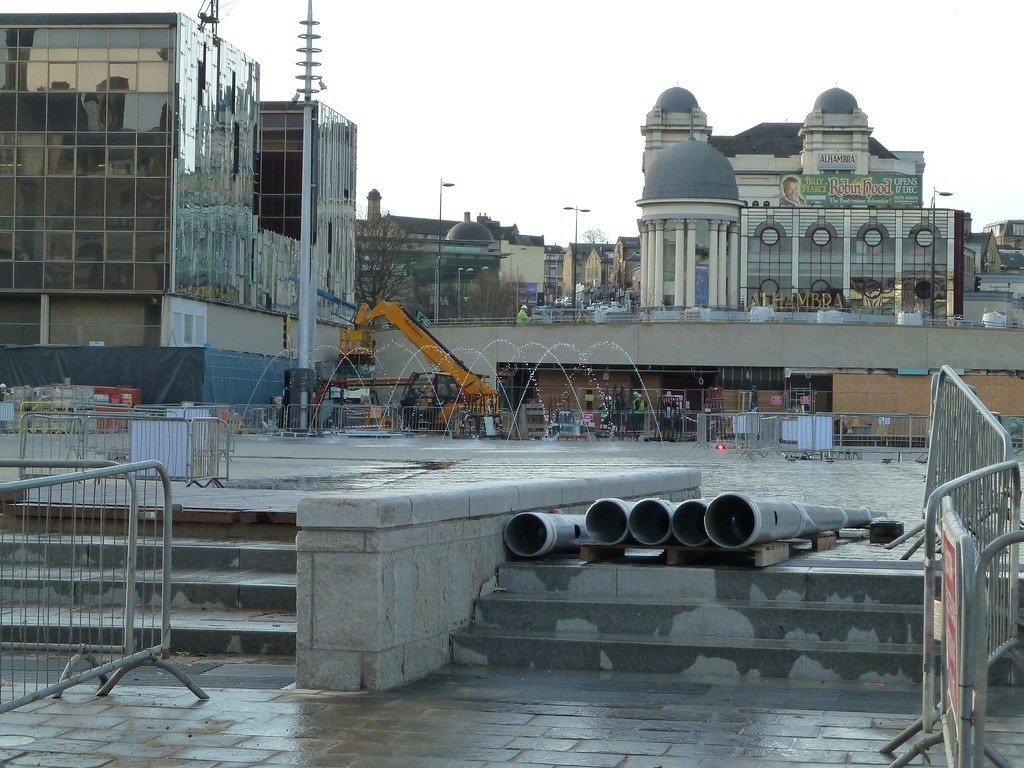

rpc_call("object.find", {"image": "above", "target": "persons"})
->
[779,177,805,205]
[638,394,648,431]
[659,390,677,438]
[598,395,613,424]
[516,304,529,323]
[615,393,628,426]
[631,392,639,430]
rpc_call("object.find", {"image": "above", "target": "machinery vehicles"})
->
[338,298,498,436]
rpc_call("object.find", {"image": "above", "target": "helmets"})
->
[521,305,527,309]
[638,393,642,397]
[0,383,6,387]
[606,396,613,401]
[633,391,638,394]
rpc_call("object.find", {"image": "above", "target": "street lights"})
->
[564,205,591,320]
[432,177,457,324]
[931,185,954,318]
